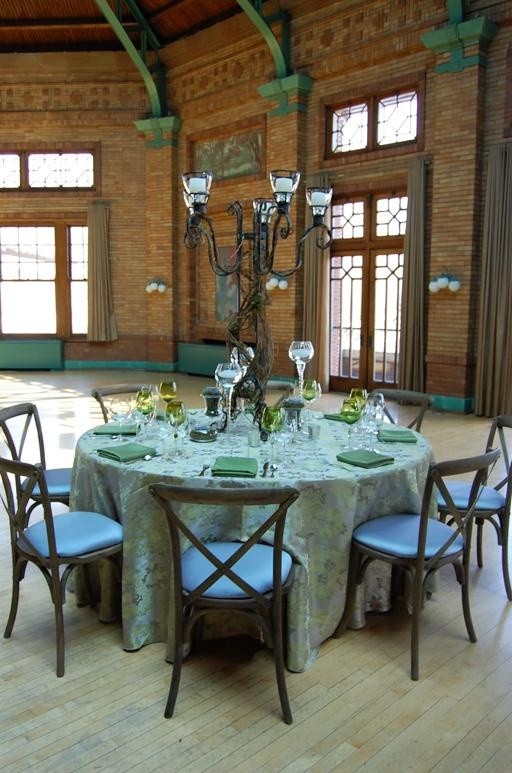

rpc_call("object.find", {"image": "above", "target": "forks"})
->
[199,459,211,476]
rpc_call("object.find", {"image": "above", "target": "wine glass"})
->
[109,339,386,465]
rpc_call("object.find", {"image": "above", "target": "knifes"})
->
[262,462,269,477]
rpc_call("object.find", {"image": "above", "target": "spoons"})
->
[270,464,277,477]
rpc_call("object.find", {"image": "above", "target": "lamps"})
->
[181,167,333,226]
[427,272,460,293]
[263,273,288,291]
[143,278,167,294]
